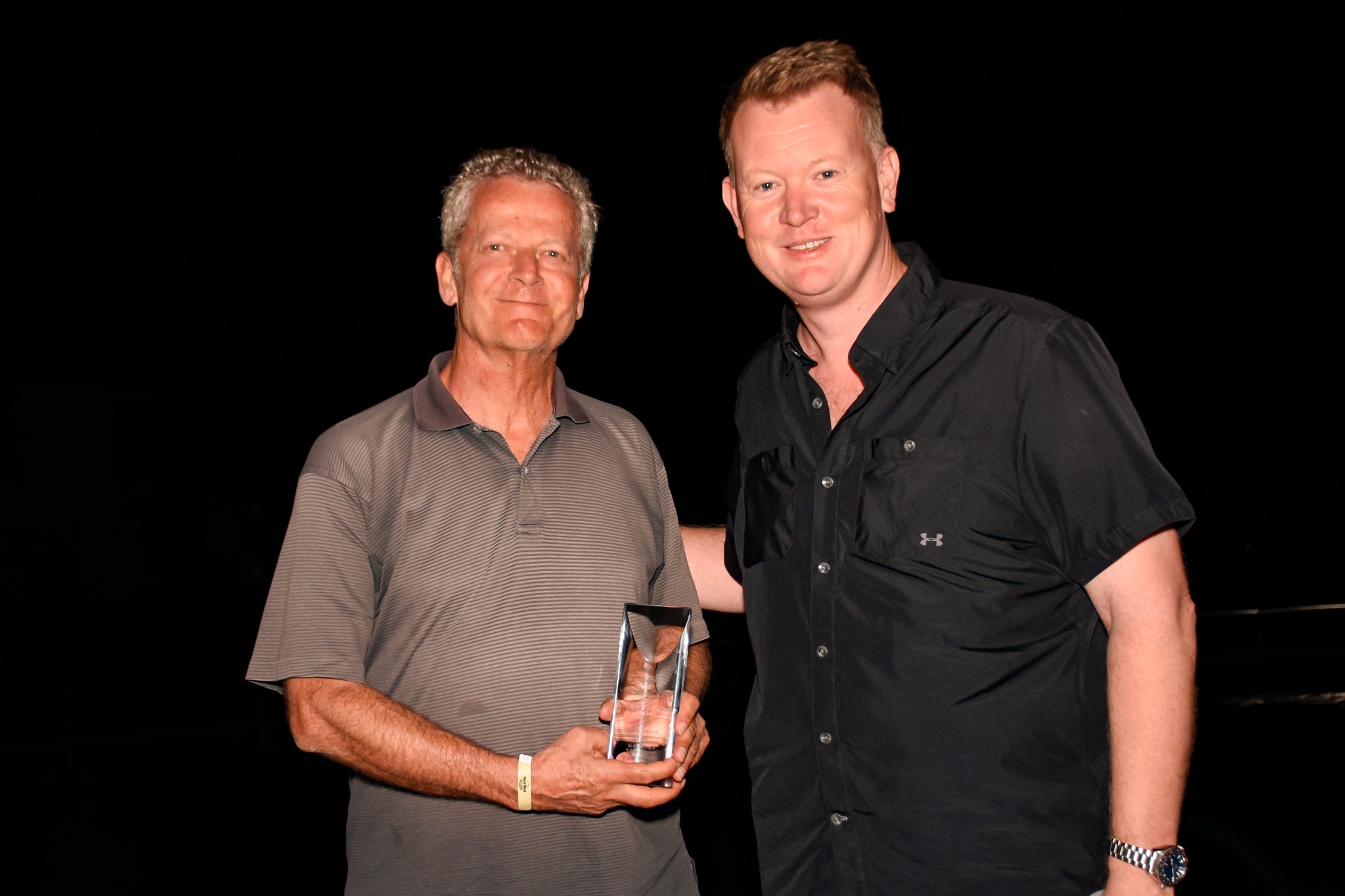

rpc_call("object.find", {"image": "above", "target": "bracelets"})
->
[516,753,532,811]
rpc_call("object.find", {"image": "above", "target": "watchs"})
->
[1107,837,1189,889]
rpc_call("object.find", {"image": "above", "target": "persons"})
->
[242,144,712,896]
[677,41,1197,895]
